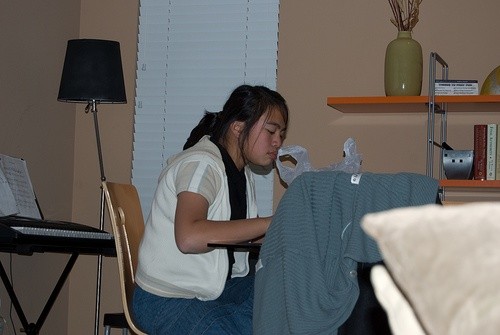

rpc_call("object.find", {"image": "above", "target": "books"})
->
[435,80,478,95]
[474,125,500,180]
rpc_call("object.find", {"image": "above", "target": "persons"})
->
[133,85,289,335]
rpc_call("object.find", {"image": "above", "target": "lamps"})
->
[57,39,127,335]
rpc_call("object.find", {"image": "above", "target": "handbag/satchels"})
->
[276,137,363,187]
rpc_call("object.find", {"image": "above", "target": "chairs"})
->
[101,181,149,335]
[299,171,443,335]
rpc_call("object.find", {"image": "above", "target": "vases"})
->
[384,30,423,96]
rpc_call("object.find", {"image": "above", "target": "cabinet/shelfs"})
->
[327,52,500,202]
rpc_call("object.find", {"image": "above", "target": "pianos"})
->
[0,214,118,335]
